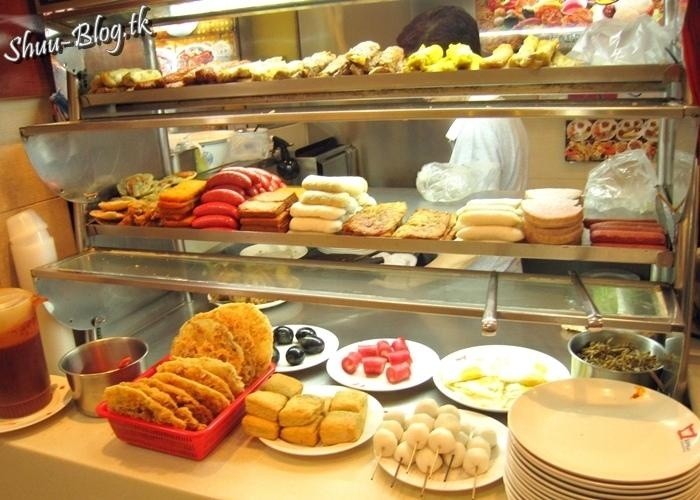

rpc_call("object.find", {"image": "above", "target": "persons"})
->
[396,6,528,273]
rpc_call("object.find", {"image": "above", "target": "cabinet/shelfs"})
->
[670,157,700,391]
[17,58,700,389]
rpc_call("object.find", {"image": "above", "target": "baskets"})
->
[95,355,276,460]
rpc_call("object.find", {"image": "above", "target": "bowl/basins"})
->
[59,336,149,419]
[567,329,669,384]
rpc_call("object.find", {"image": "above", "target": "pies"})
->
[90,171,206,227]
[104,303,274,430]
[343,203,457,241]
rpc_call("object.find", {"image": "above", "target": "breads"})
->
[242,373,368,446]
[525,187,584,244]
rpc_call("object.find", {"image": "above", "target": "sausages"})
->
[192,166,284,230]
[584,219,665,249]
[341,339,412,384]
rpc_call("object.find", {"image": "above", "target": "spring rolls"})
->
[289,175,376,233]
[455,198,524,243]
[90,36,559,93]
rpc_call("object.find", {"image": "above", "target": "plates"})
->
[258,384,384,456]
[372,408,506,493]
[238,242,310,260]
[326,337,439,393]
[1,370,72,435]
[504,371,700,499]
[566,118,660,163]
[316,243,374,254]
[435,339,567,412]
[207,289,286,312]
[270,323,338,371]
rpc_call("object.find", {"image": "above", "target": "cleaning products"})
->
[270,135,299,180]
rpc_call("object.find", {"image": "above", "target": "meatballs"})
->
[376,399,496,476]
